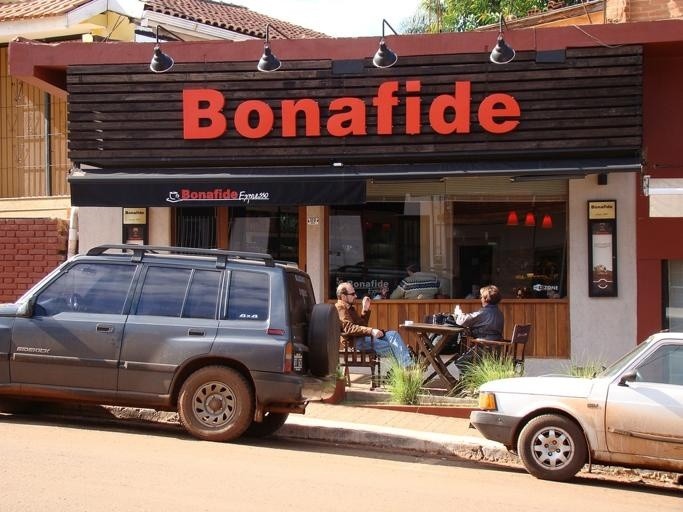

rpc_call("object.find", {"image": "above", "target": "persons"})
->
[464,284,484,301]
[389,263,440,300]
[372,283,390,301]
[335,282,418,372]
[452,285,503,377]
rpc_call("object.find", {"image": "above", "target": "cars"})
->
[470,332,683,480]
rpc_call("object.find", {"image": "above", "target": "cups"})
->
[362,296,370,312]
[404,320,414,327]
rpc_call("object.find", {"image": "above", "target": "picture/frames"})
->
[587,199,617,298]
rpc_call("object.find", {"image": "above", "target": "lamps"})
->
[372,19,398,69]
[489,12,516,64]
[505,204,553,229]
[150,25,185,74]
[257,23,293,72]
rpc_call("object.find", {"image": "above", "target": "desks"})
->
[398,323,467,391]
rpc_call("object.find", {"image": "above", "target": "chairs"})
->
[471,322,531,368]
[339,329,388,390]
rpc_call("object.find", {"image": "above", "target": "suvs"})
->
[329,259,407,298]
[0,243,340,443]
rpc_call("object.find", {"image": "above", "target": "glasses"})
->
[347,292,356,296]
